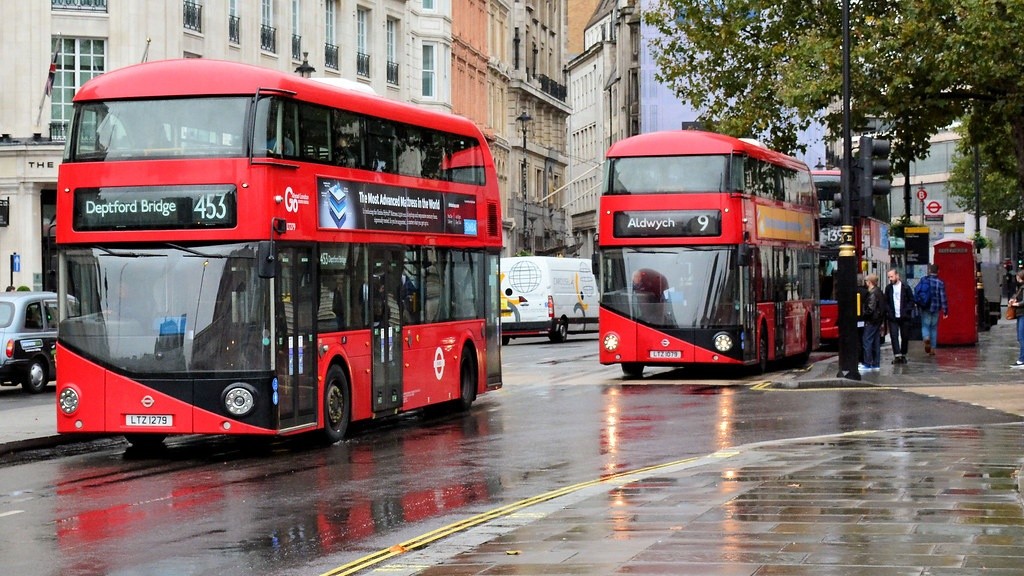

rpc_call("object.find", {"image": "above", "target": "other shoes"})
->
[929,349,935,355]
[1016,361,1024,364]
[901,356,907,364]
[892,357,901,364]
[857,363,880,371]
[925,336,931,353]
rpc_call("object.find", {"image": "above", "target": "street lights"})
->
[515,111,533,252]
[189,259,210,370]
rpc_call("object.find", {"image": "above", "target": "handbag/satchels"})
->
[1006,306,1015,320]
[917,275,931,309]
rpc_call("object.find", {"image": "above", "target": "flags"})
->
[45,41,61,97]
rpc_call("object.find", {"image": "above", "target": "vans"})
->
[492,255,599,346]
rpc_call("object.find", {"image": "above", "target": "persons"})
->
[885,269,916,365]
[858,274,886,371]
[914,263,949,358]
[267,115,294,155]
[1008,269,1024,368]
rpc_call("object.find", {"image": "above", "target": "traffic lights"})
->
[856,134,893,219]
[1016,251,1024,270]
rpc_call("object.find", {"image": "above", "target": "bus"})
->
[589,129,820,376]
[805,170,893,344]
[46,57,505,449]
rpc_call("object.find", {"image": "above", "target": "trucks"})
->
[928,211,1014,331]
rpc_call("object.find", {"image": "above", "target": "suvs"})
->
[0,290,81,395]
